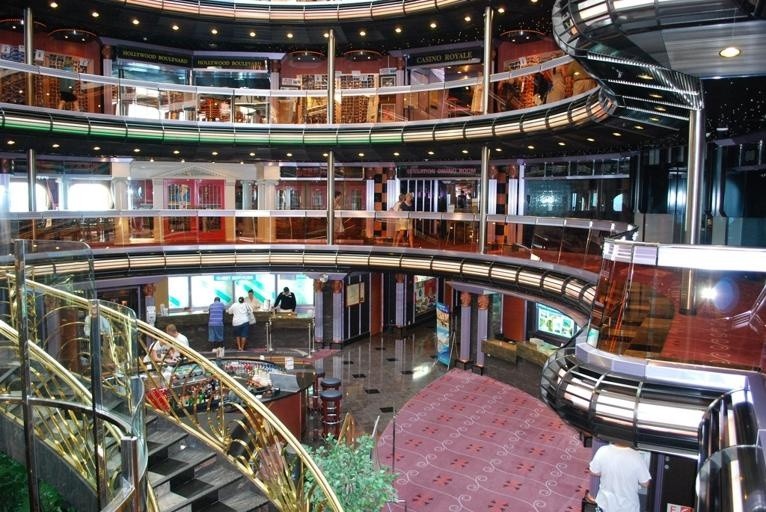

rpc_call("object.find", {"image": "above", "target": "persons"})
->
[568,60,596,96]
[334,192,344,243]
[254,110,262,123]
[393,192,409,247]
[588,440,652,512]
[395,193,414,248]
[58,57,80,112]
[83,287,296,364]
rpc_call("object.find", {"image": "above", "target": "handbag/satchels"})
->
[248,312,256,325]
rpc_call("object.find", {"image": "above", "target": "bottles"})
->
[159,304,168,317]
[173,379,239,411]
[239,362,282,381]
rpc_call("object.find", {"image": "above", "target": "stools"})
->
[321,378,341,389]
[319,391,342,439]
[305,371,324,414]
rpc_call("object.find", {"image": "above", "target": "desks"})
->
[481,340,517,366]
[520,342,559,366]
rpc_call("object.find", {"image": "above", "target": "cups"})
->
[215,348,224,359]
[285,357,294,371]
